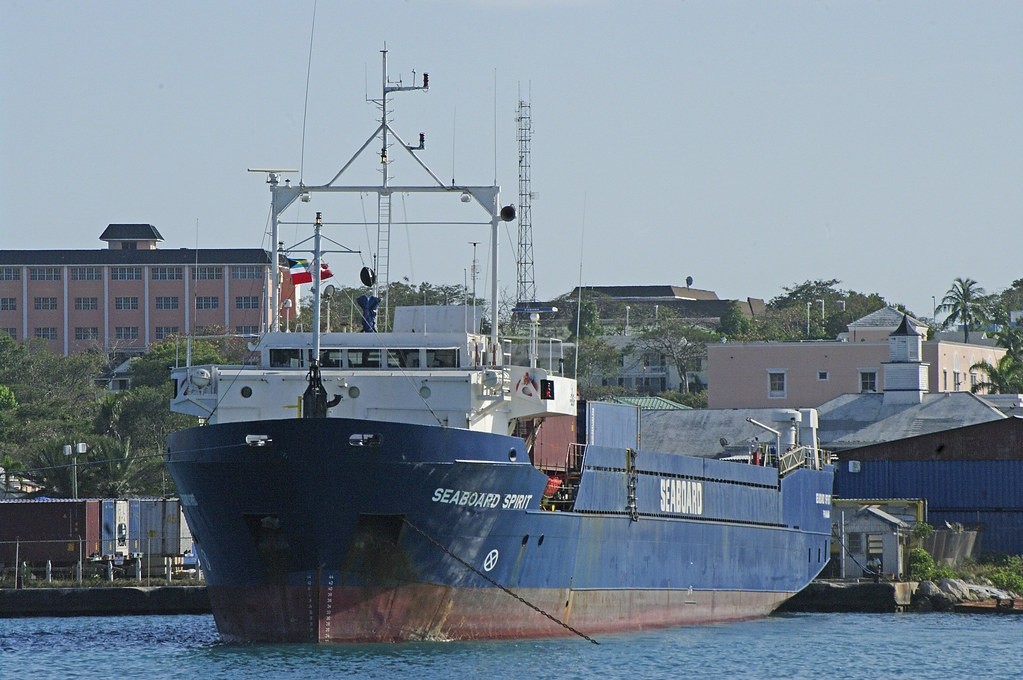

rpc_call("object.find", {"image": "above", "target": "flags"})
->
[288,258,333,285]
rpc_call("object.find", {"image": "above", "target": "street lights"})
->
[64,439,87,500]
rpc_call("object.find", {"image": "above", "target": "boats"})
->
[167,38,836,649]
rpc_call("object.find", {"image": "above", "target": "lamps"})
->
[460,192,471,202]
[301,194,311,203]
